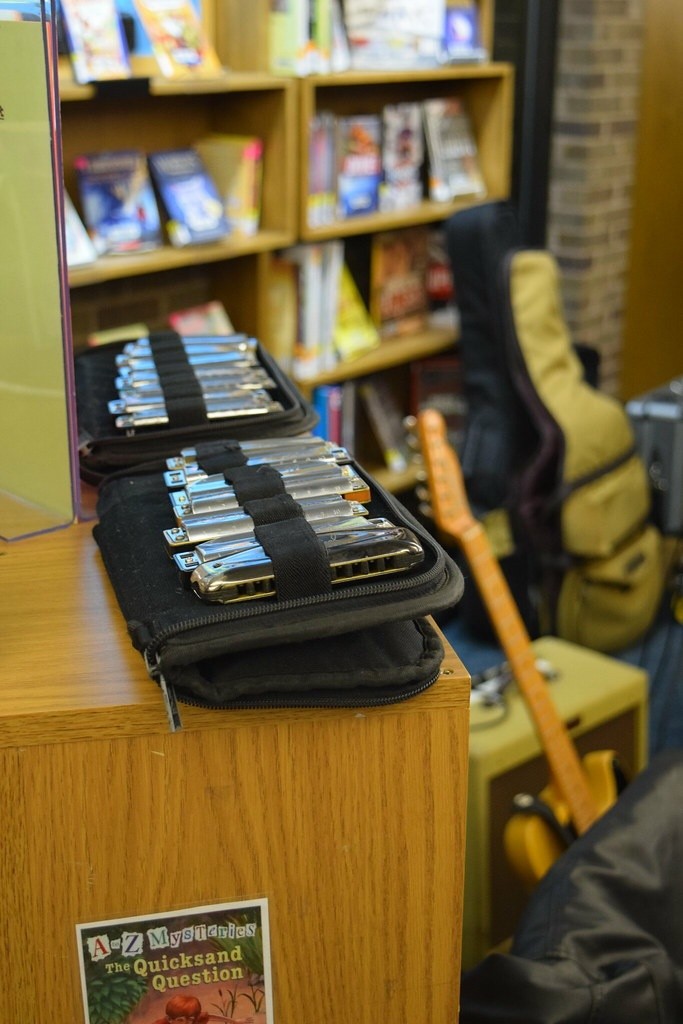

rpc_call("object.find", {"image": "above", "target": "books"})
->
[76,133,263,255]
[307,96,485,228]
[269,0,485,78]
[60,0,221,85]
[291,224,429,380]
[312,374,415,470]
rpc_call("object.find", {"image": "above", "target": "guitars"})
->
[404,409,630,889]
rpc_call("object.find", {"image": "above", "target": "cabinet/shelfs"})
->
[0,0,517,1024]
